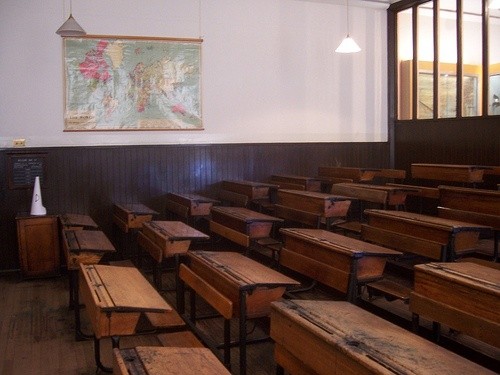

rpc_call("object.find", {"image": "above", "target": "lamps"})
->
[55,0,87,38]
[335,1,362,55]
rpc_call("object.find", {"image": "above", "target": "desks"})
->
[271,301,496,375]
[113,348,233,375]
[169,192,215,223]
[113,204,159,243]
[412,162,500,186]
[14,210,61,282]
[222,179,274,210]
[360,207,495,263]
[79,262,172,370]
[412,261,500,349]
[180,250,300,369]
[210,204,282,250]
[333,182,418,211]
[320,166,409,185]
[274,188,358,233]
[275,174,327,192]
[439,186,500,231]
[138,221,210,290]
[279,230,402,303]
[60,214,103,284]
[63,229,116,375]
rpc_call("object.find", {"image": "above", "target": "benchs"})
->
[331,184,420,237]
[278,247,355,316]
[385,184,440,215]
[158,200,190,223]
[103,214,130,243]
[146,264,234,368]
[406,291,500,372]
[133,229,164,285]
[360,224,447,333]
[436,206,500,261]
[200,220,249,258]
[255,205,320,263]
[247,181,305,212]
